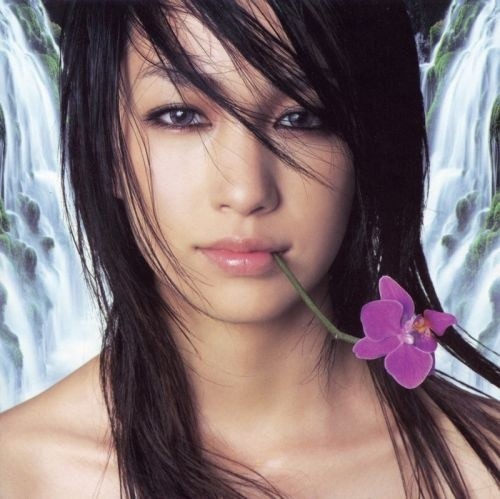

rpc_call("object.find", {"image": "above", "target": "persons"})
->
[1,0,499,499]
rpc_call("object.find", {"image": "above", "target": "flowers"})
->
[270,252,455,391]
